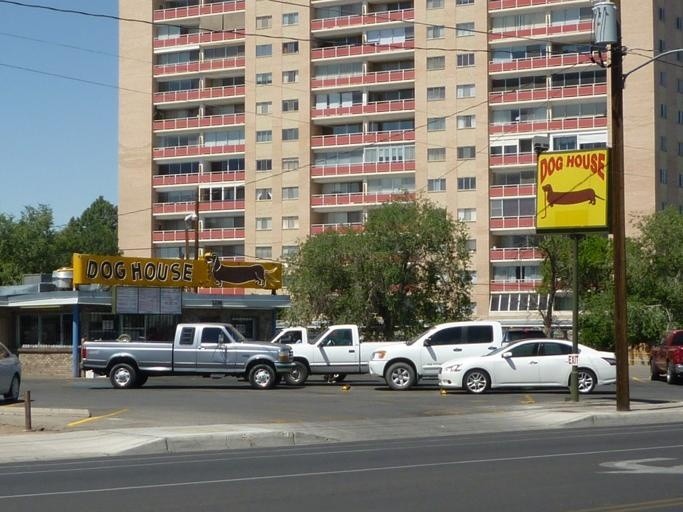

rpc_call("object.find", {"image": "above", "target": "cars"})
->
[0,342,22,403]
[438,338,617,394]
[649,329,683,384]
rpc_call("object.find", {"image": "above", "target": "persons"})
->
[292,331,301,343]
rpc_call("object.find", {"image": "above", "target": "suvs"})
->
[504,328,548,342]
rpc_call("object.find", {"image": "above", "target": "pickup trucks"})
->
[369,320,511,391]
[267,326,323,348]
[80,322,297,391]
[284,325,410,387]
